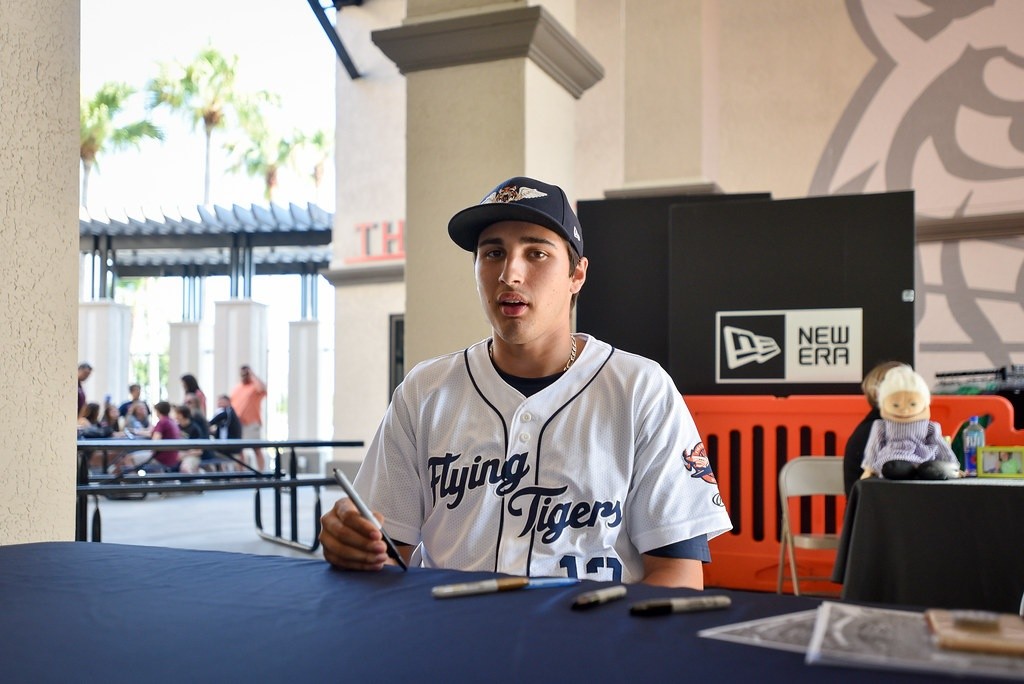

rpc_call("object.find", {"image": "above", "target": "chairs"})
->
[777,456,847,597]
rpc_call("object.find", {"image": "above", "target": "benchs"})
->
[86,470,283,481]
[123,459,231,472]
[76,478,340,495]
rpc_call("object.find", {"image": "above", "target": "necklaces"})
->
[488,335,576,372]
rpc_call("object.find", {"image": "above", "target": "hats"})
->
[448,177,584,260]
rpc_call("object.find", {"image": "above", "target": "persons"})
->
[78,395,241,483]
[843,360,910,498]
[318,177,734,591]
[860,365,964,480]
[118,385,150,418]
[77,363,92,411]
[994,451,1019,474]
[230,366,267,479]
[182,375,206,417]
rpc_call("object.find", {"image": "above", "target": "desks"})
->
[0,542,1024,684]
[75,440,365,554]
[90,434,228,501]
[832,478,1024,616]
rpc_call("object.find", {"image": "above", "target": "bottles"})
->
[962,416,986,477]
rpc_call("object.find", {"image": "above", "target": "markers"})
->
[332,467,408,572]
[431,576,529,599]
[568,584,628,612]
[629,594,732,618]
[523,577,581,590]
